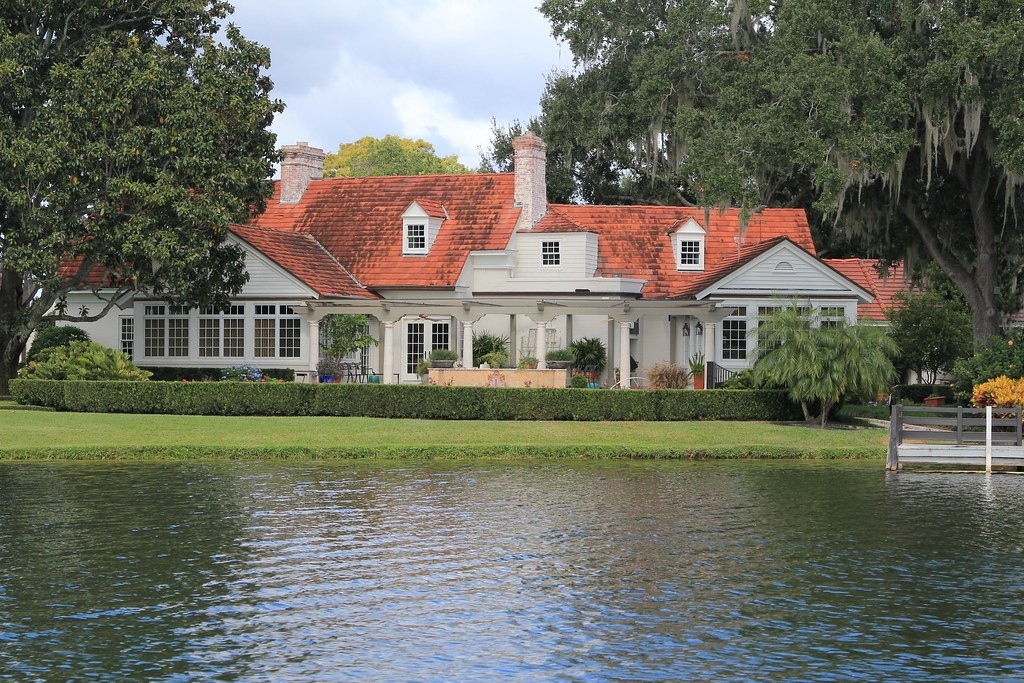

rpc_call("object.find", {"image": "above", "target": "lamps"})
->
[696,322,702,335]
[682,323,689,336]
[536,301,544,311]
[623,304,630,312]
[462,303,469,311]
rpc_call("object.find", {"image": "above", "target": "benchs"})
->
[340,362,375,384]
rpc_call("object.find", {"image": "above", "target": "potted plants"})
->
[684,350,704,389]
[570,336,607,379]
[545,349,572,369]
[518,349,540,369]
[924,393,947,407]
[416,358,428,384]
[429,348,458,368]
